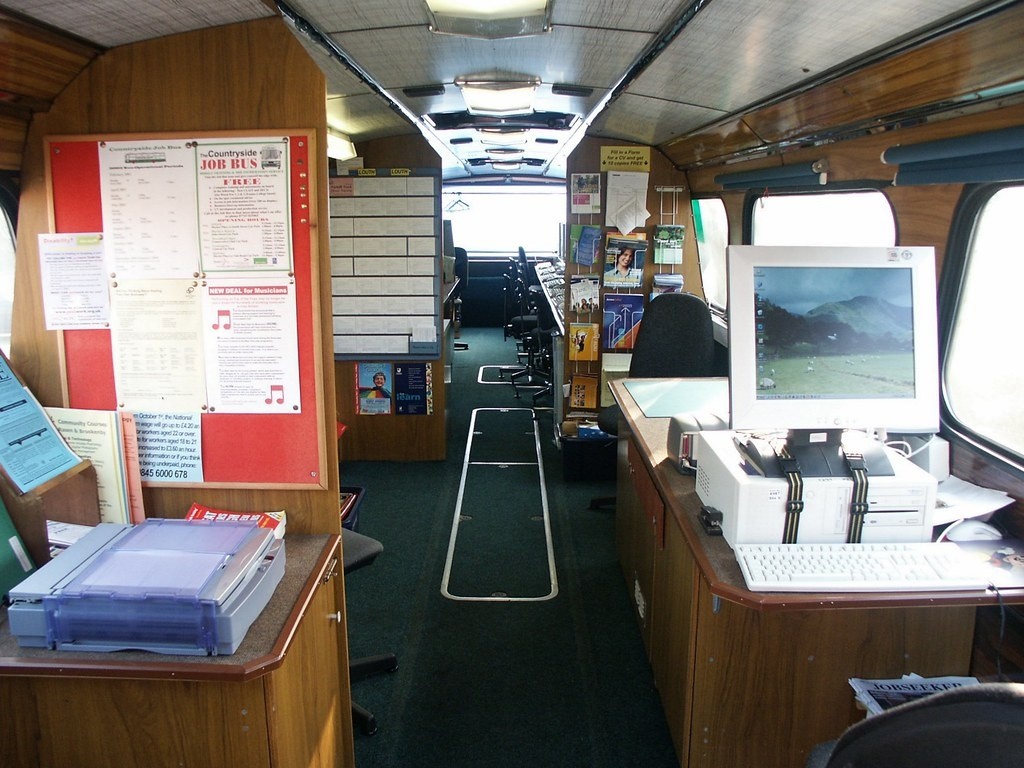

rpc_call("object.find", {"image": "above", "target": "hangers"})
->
[444,192,470,211]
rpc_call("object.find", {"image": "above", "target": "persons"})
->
[575,297,598,313]
[573,384,587,408]
[573,330,588,352]
[373,372,386,388]
[604,247,642,287]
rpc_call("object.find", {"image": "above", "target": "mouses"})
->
[944,519,1003,541]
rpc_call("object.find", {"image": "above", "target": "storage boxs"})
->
[340,486,365,533]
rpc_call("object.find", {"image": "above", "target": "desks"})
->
[443,275,461,305]
[534,263,566,336]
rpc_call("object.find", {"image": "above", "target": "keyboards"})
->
[733,542,986,592]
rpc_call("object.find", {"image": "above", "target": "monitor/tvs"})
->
[694,245,940,476]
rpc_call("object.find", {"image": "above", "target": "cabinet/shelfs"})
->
[0,460,343,768]
[607,379,1024,768]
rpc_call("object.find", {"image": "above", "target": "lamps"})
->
[326,128,357,161]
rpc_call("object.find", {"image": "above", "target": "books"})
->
[183,499,287,546]
[603,237,648,288]
[601,353,632,408]
[568,374,599,409]
[649,274,684,302]
[606,230,648,249]
[653,224,685,265]
[569,322,599,360]
[602,292,645,348]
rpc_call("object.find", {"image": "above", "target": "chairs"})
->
[450,247,469,338]
[591,294,713,508]
[807,683,1024,768]
[500,247,558,405]
[341,528,399,736]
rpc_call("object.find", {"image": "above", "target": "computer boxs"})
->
[696,430,937,550]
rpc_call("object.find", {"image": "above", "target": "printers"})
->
[7,521,286,657]
